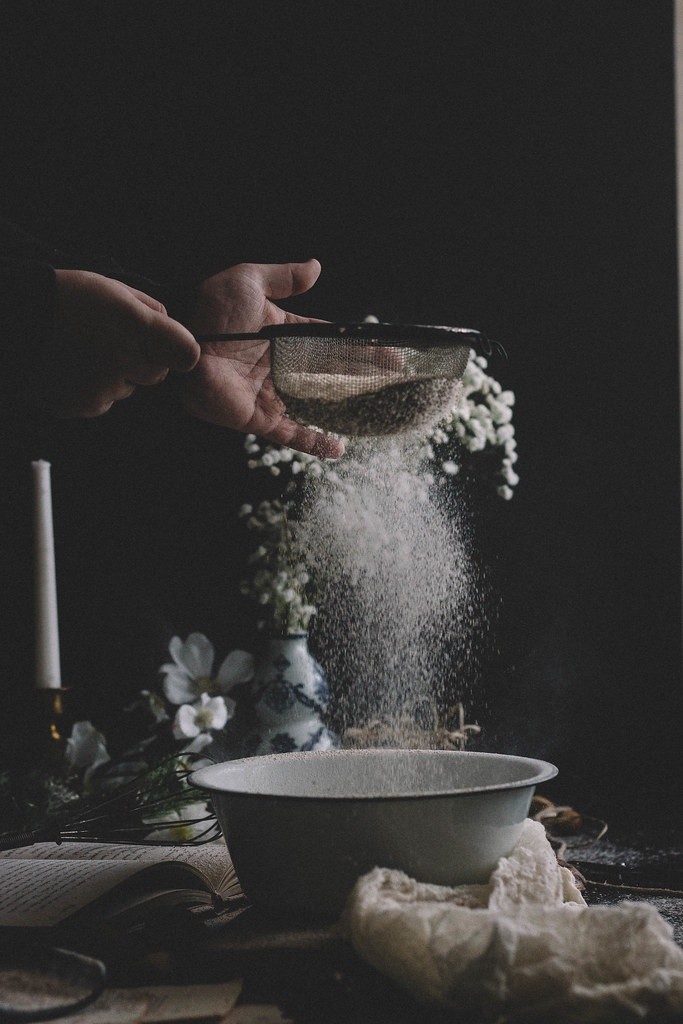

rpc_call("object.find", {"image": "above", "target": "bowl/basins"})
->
[187,750,558,926]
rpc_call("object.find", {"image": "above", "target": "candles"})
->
[30,459,62,689]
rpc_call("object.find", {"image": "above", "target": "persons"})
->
[1,256,405,462]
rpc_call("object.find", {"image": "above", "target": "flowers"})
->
[62,631,256,837]
